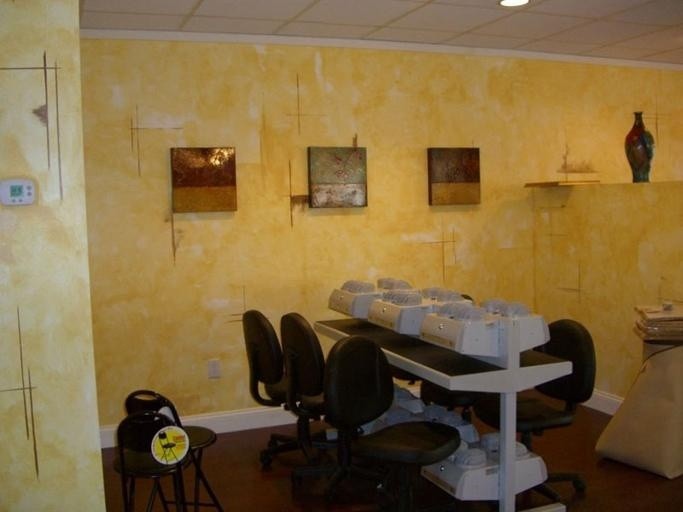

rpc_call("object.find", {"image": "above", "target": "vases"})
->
[624,111,656,184]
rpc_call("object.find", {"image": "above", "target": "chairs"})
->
[473,318,591,502]
[124,389,217,510]
[117,411,226,511]
[419,387,491,413]
[390,294,476,385]
[278,311,379,491]
[240,310,343,482]
[325,335,461,509]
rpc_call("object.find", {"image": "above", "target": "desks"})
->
[314,276,572,510]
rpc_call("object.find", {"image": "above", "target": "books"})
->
[630,300,681,344]
